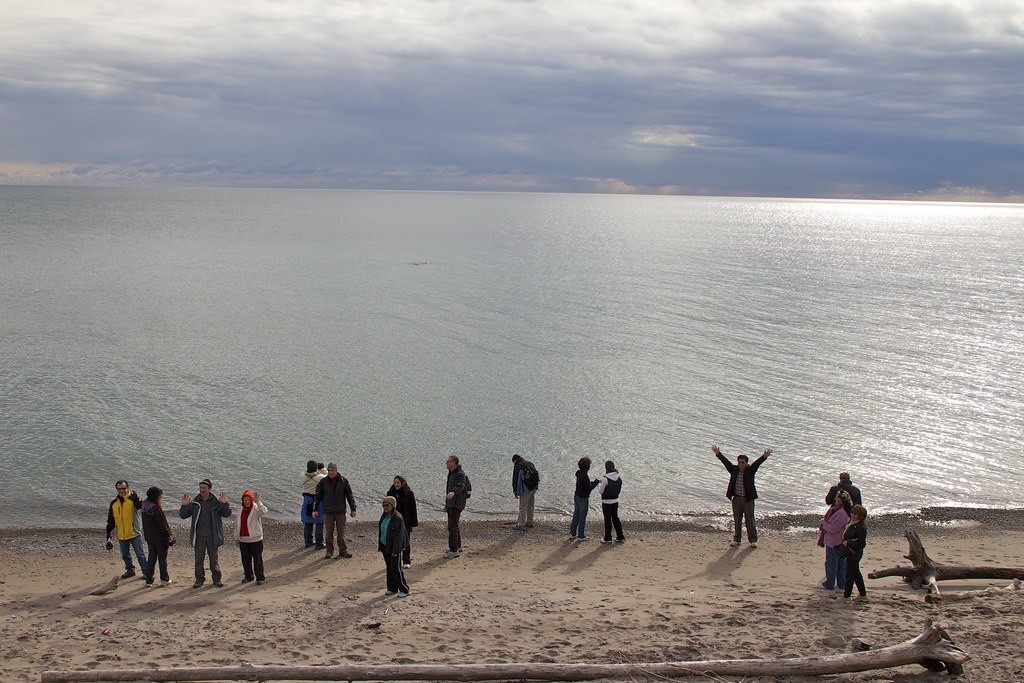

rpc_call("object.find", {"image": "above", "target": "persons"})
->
[300,459,329,550]
[442,455,472,559]
[711,445,774,548]
[595,460,625,542]
[311,463,357,559]
[376,496,412,598]
[512,454,540,530]
[385,475,418,569]
[141,486,176,587]
[568,456,602,541]
[234,490,268,586]
[818,472,869,603]
[179,479,233,588]
[105,480,148,579]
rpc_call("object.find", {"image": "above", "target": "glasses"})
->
[447,461,457,463]
[117,488,127,490]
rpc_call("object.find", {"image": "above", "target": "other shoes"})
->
[601,537,612,543]
[751,542,757,547]
[614,537,623,541]
[578,537,590,540]
[818,584,826,588]
[305,540,326,550]
[325,553,332,558]
[513,525,526,531]
[443,548,463,559]
[731,540,741,546]
[340,552,353,558]
[569,533,579,539]
[120,570,265,588]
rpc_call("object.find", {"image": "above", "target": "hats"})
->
[383,496,397,508]
[199,479,211,488]
[146,486,163,500]
[307,460,318,470]
[241,490,253,505]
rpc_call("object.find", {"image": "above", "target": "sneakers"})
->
[837,596,852,604]
[385,590,411,597]
[403,563,410,569]
[854,595,870,603]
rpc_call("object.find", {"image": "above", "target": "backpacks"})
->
[454,472,472,498]
[518,463,539,489]
